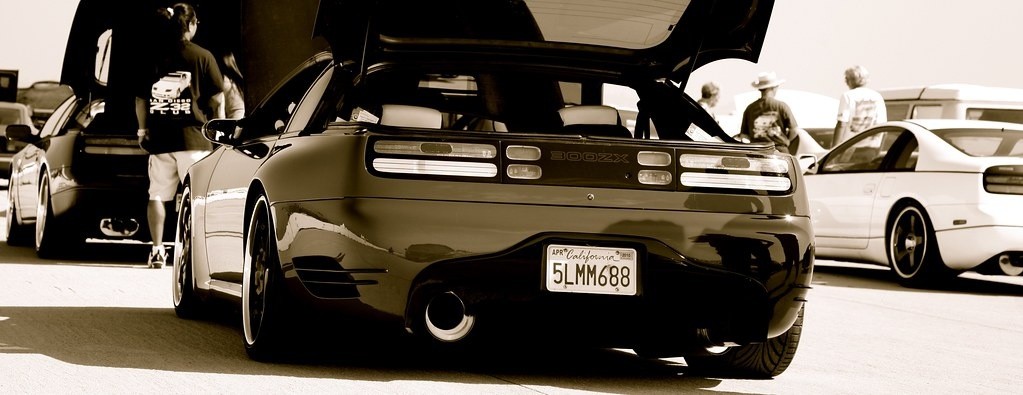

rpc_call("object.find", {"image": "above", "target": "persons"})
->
[740,75,800,156]
[831,66,887,164]
[698,83,721,124]
[134,5,245,268]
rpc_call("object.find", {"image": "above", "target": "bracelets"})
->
[137,129,148,136]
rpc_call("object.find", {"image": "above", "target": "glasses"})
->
[192,20,200,26]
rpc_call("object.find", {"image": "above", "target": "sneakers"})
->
[148,250,167,269]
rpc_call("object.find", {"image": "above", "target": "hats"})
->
[751,72,781,89]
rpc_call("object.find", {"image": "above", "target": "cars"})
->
[170,0,819,380]
[796,85,1023,289]
[0,67,185,259]
